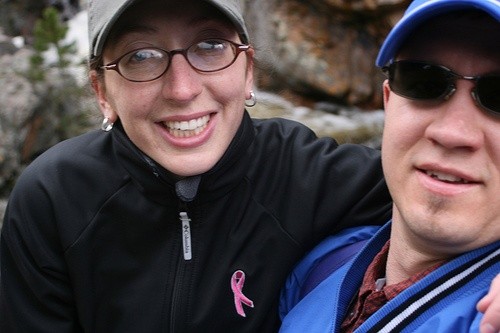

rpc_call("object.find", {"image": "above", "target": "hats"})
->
[375,0,500,68]
[87,0,251,57]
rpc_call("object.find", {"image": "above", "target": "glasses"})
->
[379,59,500,117]
[98,38,251,83]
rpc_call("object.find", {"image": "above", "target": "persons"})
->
[272,0,500,333]
[0,0,500,332]
